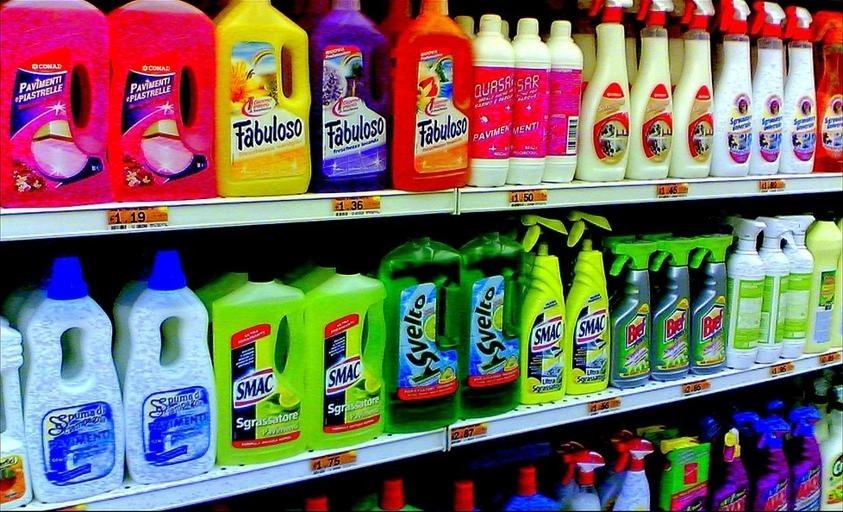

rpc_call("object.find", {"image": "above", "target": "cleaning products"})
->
[455,0,843,188]
[305,368,843,512]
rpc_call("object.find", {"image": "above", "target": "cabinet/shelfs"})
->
[1,170,842,512]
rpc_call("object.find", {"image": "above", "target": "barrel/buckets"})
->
[195,257,251,357]
[0,0,112,209]
[112,248,217,484]
[106,0,217,203]
[378,209,843,434]
[212,0,312,198]
[308,0,393,194]
[288,249,340,295]
[0,317,33,510]
[212,255,306,467]
[389,0,473,192]
[17,256,125,503]
[304,248,386,451]
